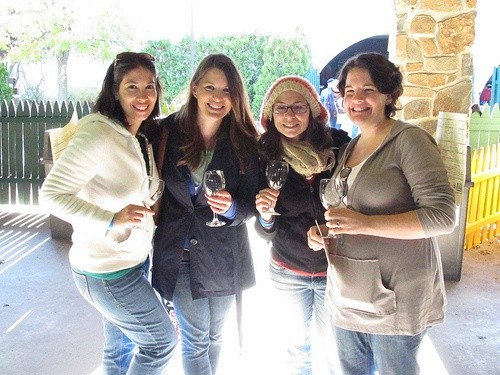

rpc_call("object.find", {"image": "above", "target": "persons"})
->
[306,52,456,375]
[38,52,180,375]
[253,76,353,375]
[151,54,260,375]
[480,84,492,106]
[471,103,483,118]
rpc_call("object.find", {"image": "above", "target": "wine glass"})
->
[129,176,165,233]
[261,161,289,216]
[319,179,344,239]
[203,170,226,227]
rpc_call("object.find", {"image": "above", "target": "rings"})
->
[336,220,340,227]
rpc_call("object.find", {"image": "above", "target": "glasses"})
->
[337,167,353,196]
[113,52,155,73]
[272,104,309,115]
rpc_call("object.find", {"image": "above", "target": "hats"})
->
[261,76,330,132]
